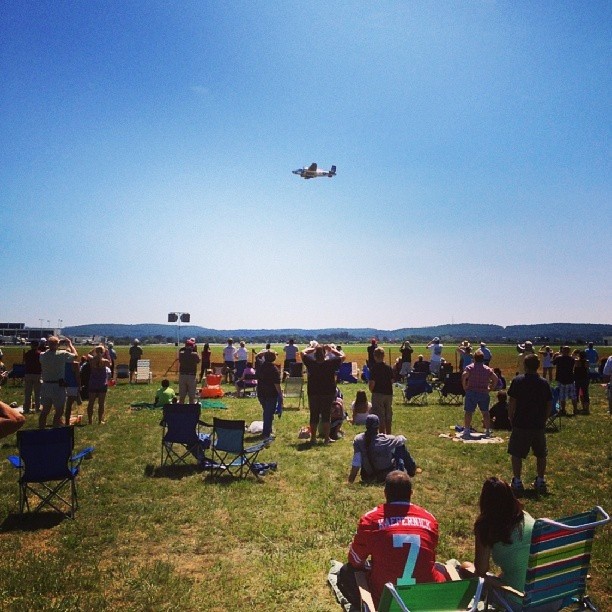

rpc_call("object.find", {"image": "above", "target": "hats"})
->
[479,342,486,347]
[371,337,377,345]
[186,340,195,348]
[432,337,440,341]
[364,414,379,428]
[39,337,47,345]
[461,340,470,346]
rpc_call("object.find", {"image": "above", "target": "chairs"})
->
[239,374,257,394]
[354,565,484,612]
[438,373,462,404]
[337,363,352,383]
[546,386,561,429]
[10,426,90,520]
[415,362,430,379]
[159,404,212,470]
[441,363,453,373]
[466,507,609,612]
[134,360,152,383]
[282,377,304,409]
[213,418,275,483]
[401,372,432,405]
[401,362,412,381]
[115,365,130,383]
[11,363,25,384]
[284,362,303,377]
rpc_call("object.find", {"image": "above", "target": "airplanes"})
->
[291,162,337,181]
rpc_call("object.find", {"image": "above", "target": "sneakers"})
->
[534,477,545,488]
[460,428,470,435]
[511,477,522,488]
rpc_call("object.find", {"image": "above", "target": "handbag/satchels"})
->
[221,363,230,375]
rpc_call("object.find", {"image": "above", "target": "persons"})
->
[85,345,113,426]
[198,342,212,383]
[79,356,86,366]
[602,354,612,414]
[441,356,447,363]
[335,469,451,612]
[0,394,27,437]
[425,337,444,374]
[456,474,538,612]
[551,345,565,359]
[221,337,237,384]
[515,340,535,374]
[457,349,499,438]
[458,340,473,371]
[63,347,84,427]
[348,389,372,426]
[392,355,404,384]
[38,335,46,352]
[574,351,590,414]
[368,345,396,435]
[176,337,201,404]
[153,377,178,409]
[549,345,578,416]
[317,397,347,440]
[584,341,600,383]
[106,341,118,380]
[232,340,248,379]
[235,361,258,396]
[476,340,493,367]
[323,343,335,358]
[128,338,144,383]
[537,345,554,380]
[598,356,609,383]
[335,344,345,355]
[346,413,417,484]
[38,336,80,429]
[455,345,475,373]
[398,340,413,382]
[572,347,581,359]
[299,342,346,445]
[282,338,299,382]
[0,347,9,383]
[106,364,111,400]
[260,343,272,363]
[502,352,556,489]
[412,354,424,371]
[366,337,378,368]
[20,339,41,413]
[256,351,284,440]
